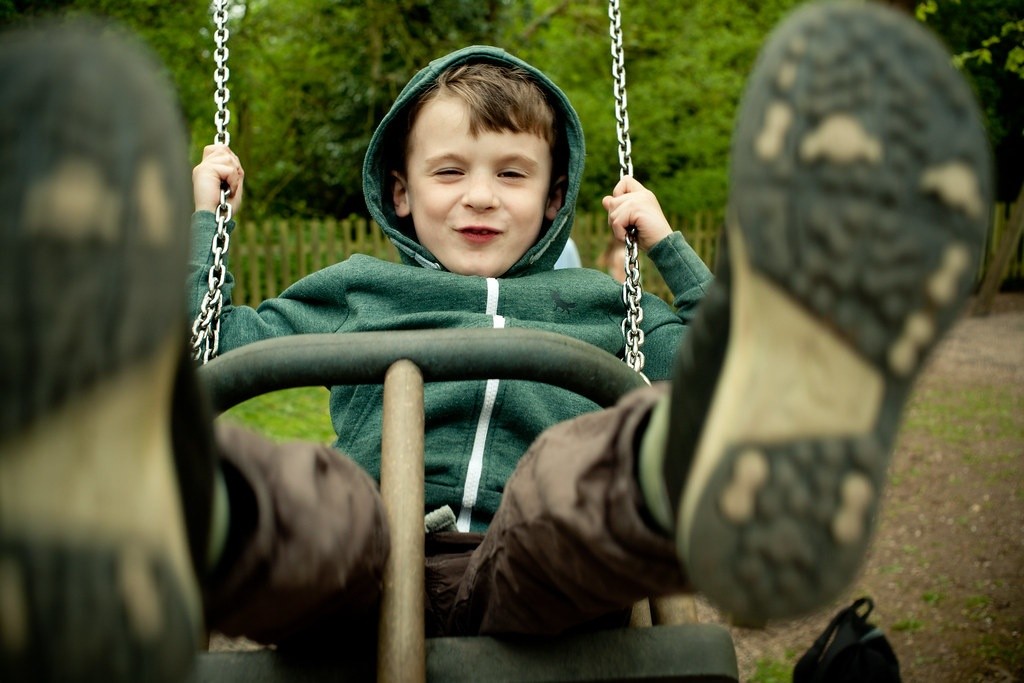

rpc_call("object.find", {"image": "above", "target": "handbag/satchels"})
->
[792,596,903,683]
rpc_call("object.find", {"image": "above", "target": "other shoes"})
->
[0,28,212,683]
[663,0,993,630]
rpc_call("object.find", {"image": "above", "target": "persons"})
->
[0,2,998,683]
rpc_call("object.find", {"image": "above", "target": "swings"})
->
[178,0,745,683]
